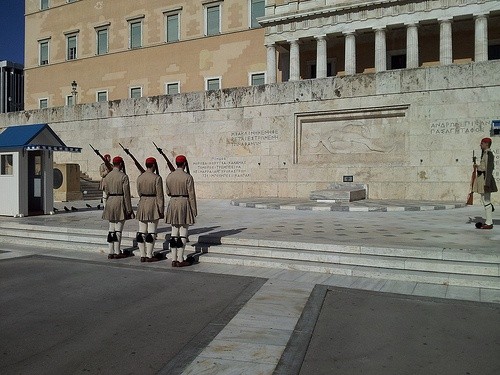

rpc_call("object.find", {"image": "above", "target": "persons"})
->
[136,157,164,262]
[165,155,198,267]
[102,156,132,259]
[99,154,113,190]
[472,137,498,229]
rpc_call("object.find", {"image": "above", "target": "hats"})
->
[176,155,186,162]
[482,138,491,143]
[113,157,121,163]
[146,158,156,164]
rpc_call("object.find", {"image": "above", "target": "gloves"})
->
[473,164,478,168]
[159,213,164,219]
[126,212,135,219]
[484,186,489,192]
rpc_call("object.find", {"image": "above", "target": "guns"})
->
[89,144,113,172]
[152,141,176,171]
[466,150,477,205]
[119,142,145,173]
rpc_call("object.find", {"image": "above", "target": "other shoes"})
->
[146,256,160,262]
[114,253,126,259]
[141,257,146,262]
[172,261,176,267]
[475,222,493,229]
[176,260,190,267]
[108,254,114,259]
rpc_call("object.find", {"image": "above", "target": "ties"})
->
[481,150,484,159]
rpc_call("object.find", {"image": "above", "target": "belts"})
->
[141,194,156,197]
[108,194,124,196]
[477,170,485,173]
[171,195,189,197]
